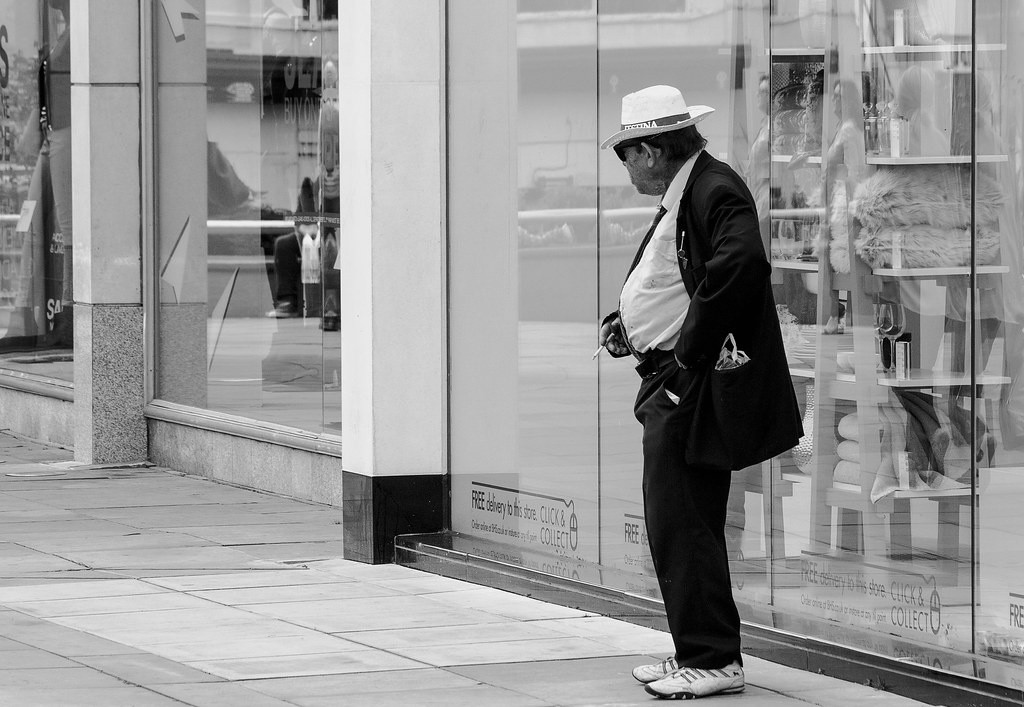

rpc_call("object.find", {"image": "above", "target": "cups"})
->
[774,217,822,263]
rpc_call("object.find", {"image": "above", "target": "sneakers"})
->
[268,302,299,318]
[645,660,746,700]
[320,316,339,331]
[632,656,679,683]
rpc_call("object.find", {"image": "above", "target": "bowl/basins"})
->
[850,354,880,374]
[837,351,851,373]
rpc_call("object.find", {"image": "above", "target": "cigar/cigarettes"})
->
[592,333,614,360]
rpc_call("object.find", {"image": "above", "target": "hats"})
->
[600,85,715,150]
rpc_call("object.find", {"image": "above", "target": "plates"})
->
[791,324,853,370]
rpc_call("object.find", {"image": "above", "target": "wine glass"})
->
[871,304,894,378]
[880,303,909,378]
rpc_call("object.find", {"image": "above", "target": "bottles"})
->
[864,102,909,158]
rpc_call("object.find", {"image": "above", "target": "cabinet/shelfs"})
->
[755,31,1012,607]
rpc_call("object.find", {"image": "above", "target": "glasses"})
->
[612,141,660,161]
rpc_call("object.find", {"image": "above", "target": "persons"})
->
[259,6,340,331]
[599,83,805,701]
[747,63,1004,493]
[0,1,73,352]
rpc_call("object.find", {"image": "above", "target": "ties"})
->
[619,204,668,362]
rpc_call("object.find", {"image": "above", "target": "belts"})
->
[634,349,676,381]
